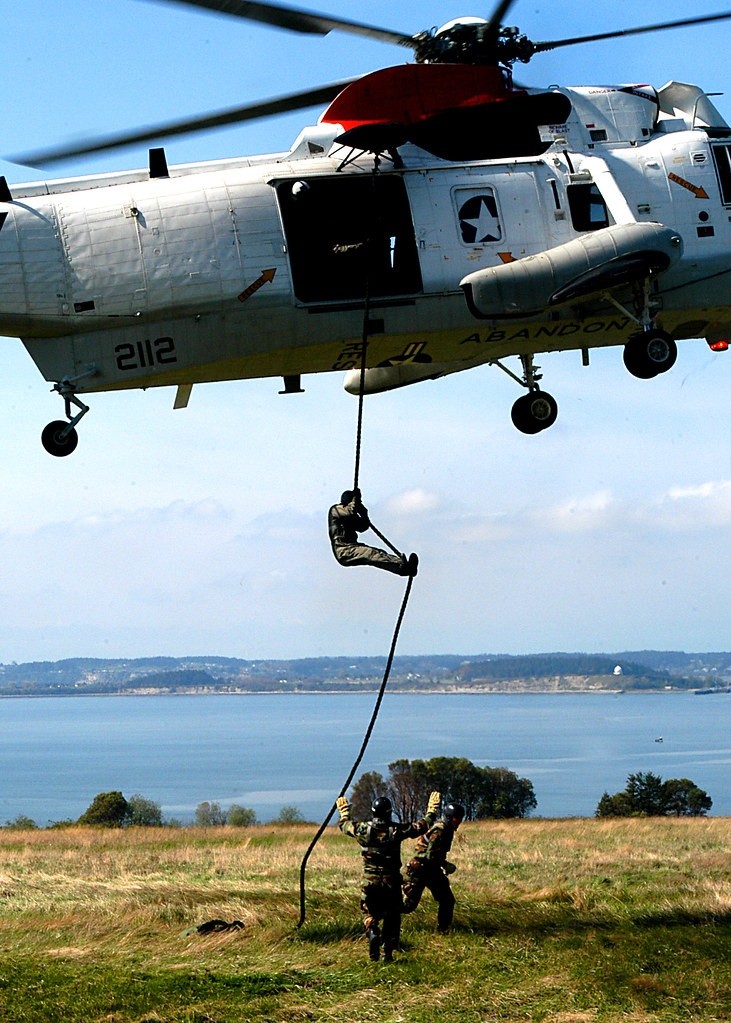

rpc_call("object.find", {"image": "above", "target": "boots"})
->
[369,926,382,961]
[381,940,396,963]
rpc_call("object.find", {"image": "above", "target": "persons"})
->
[328,489,418,578]
[335,791,443,963]
[403,803,465,931]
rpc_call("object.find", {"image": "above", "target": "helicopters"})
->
[0,1,731,458]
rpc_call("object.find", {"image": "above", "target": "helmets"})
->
[371,797,392,814]
[442,803,465,818]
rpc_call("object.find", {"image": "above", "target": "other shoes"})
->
[409,553,419,576]
[436,924,451,935]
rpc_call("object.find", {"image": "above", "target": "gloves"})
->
[427,792,441,815]
[335,797,353,822]
[445,863,457,876]
